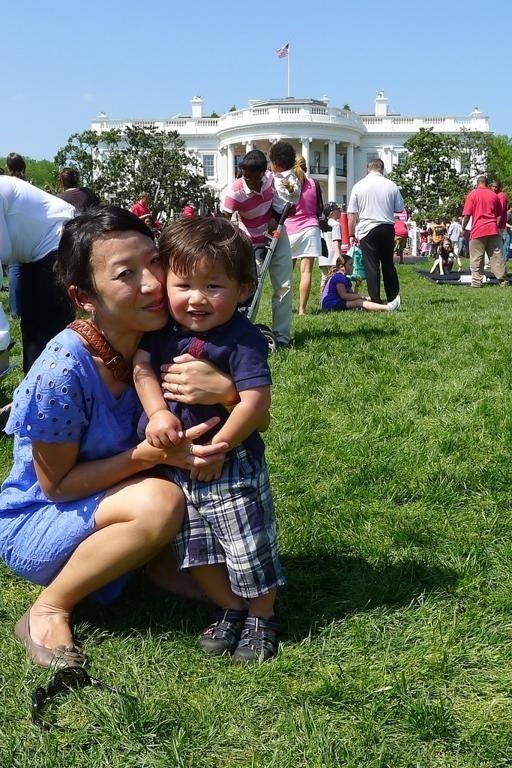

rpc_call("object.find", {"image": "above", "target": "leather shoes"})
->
[15,606,85,670]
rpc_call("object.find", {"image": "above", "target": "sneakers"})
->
[197,608,248,655]
[233,614,282,664]
[387,294,401,312]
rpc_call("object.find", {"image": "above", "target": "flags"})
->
[274,42,289,58]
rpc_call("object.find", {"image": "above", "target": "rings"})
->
[190,444,194,454]
[176,383,180,393]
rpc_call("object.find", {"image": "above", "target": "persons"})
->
[2,152,102,409]
[131,215,286,665]
[1,207,271,673]
[419,176,511,287]
[129,192,197,249]
[221,143,410,350]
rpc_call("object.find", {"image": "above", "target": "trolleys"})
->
[236,200,292,355]
